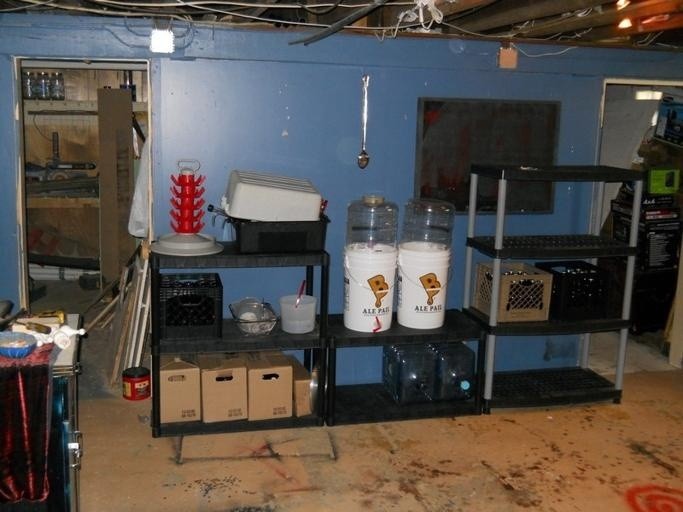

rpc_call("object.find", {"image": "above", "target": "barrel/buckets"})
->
[340,242,400,333]
[279,280,319,335]
[343,195,400,253]
[399,197,456,252]
[396,242,455,330]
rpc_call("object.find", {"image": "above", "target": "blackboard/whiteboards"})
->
[415,96,562,215]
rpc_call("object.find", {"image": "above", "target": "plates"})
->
[0,330,36,357]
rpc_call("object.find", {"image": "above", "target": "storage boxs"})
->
[159,351,310,425]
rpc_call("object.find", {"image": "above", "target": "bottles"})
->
[35,71,51,99]
[21,71,35,99]
[50,72,66,100]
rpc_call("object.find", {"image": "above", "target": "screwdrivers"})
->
[14,320,51,334]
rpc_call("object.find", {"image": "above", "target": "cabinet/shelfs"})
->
[461,164,645,417]
[0,312,83,512]
[311,308,485,427]
[150,238,329,439]
